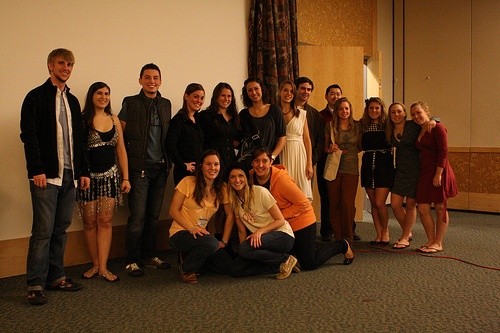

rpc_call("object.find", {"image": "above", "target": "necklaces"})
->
[283,108,294,116]
[396,133,402,140]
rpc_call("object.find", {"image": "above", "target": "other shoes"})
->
[178,251,197,283]
[321,234,333,240]
[274,255,300,279]
[353,234,361,240]
[368,241,390,249]
[343,238,355,265]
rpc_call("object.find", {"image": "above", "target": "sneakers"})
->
[48,277,83,292]
[123,263,144,277]
[26,290,47,305]
[145,257,168,269]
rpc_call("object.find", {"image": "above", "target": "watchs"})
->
[271,153,276,161]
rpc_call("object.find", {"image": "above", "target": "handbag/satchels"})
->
[323,121,343,181]
[230,124,265,163]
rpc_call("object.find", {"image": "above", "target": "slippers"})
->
[98,271,120,282]
[81,268,98,279]
[416,246,428,252]
[398,237,412,242]
[420,248,444,253]
[393,243,410,249]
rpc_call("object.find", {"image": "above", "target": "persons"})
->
[316,84,361,242]
[117,63,172,277]
[228,168,301,280]
[164,83,205,188]
[386,102,441,249]
[75,80,131,282]
[324,96,360,247]
[359,96,393,245]
[237,76,286,166]
[248,147,356,271]
[410,101,457,254]
[293,76,320,193]
[166,149,234,285]
[199,82,239,243]
[19,48,85,304]
[273,79,315,205]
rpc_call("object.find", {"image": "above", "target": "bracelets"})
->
[122,178,130,182]
[189,225,200,232]
[221,239,227,246]
